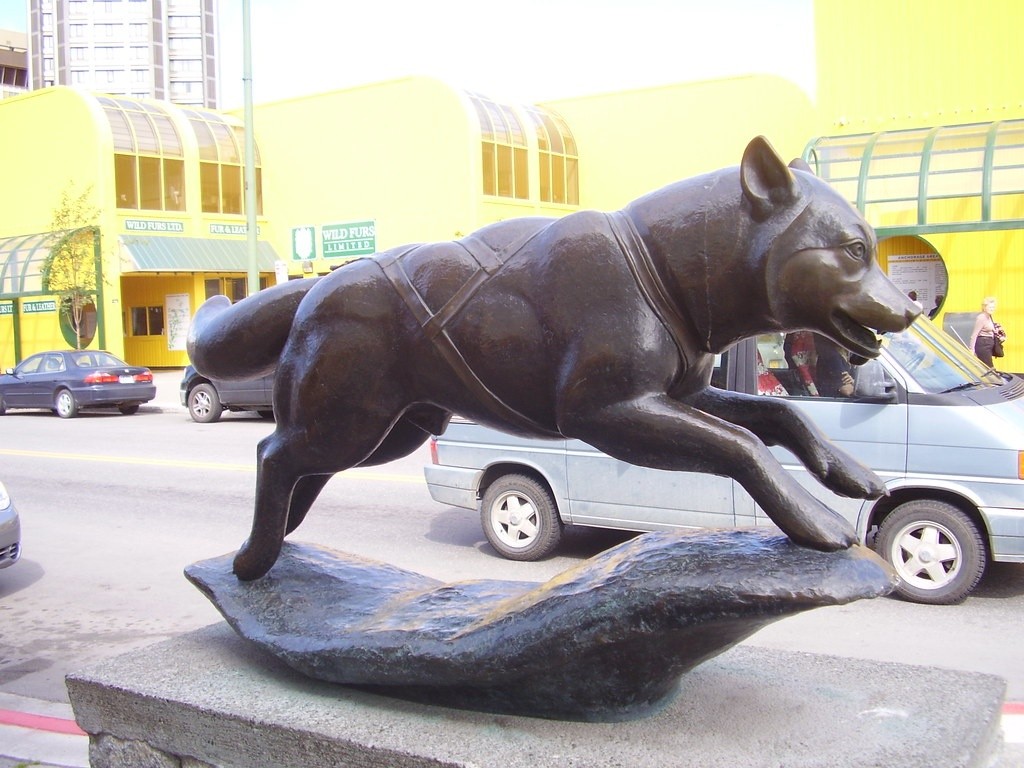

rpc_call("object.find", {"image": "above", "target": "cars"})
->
[0,349,157,419]
[178,363,275,423]
[420,311,1024,607]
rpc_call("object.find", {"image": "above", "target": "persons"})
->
[908,292,923,310]
[813,334,855,398]
[928,295,944,318]
[970,297,999,368]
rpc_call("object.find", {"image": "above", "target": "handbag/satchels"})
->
[992,334,1005,357]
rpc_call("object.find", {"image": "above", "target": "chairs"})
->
[49,359,61,371]
[756,344,788,397]
[782,333,822,398]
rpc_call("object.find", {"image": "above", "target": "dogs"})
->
[185,132,923,580]
